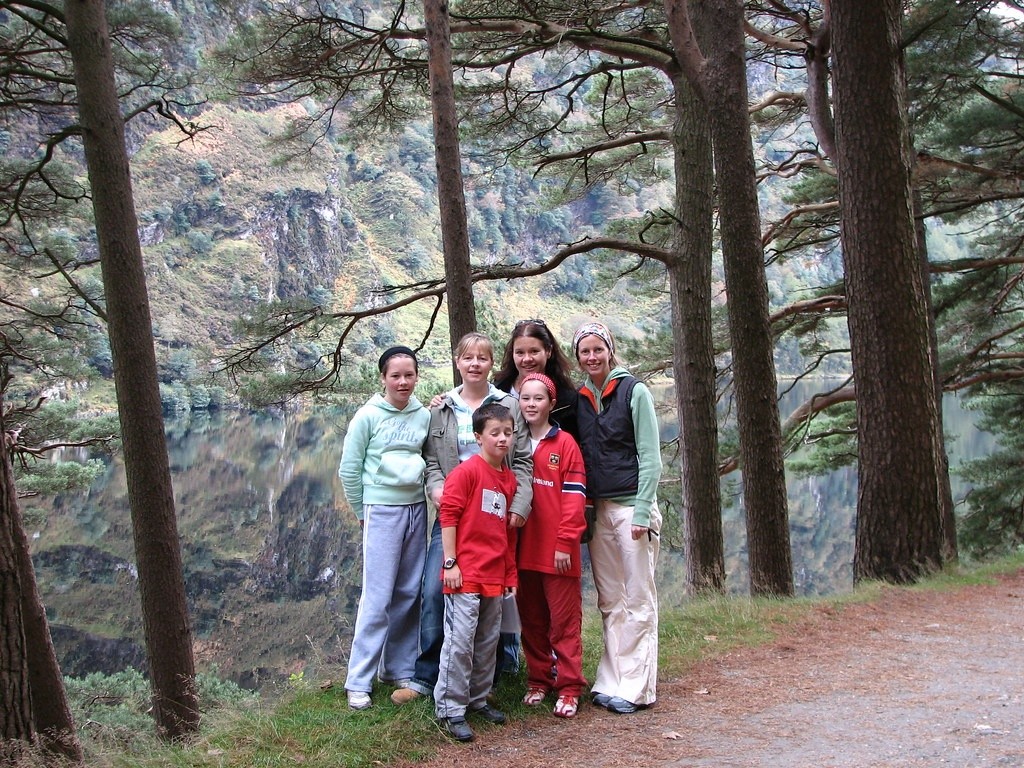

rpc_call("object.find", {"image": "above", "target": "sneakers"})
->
[607,697,650,713]
[476,705,505,723]
[443,715,473,740]
[521,686,547,705]
[553,695,579,718]
[593,694,612,706]
[347,689,371,711]
[378,675,411,689]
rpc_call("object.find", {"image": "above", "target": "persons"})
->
[434,403,519,741]
[391,319,596,705]
[339,345,432,709]
[516,372,587,719]
[572,321,663,713]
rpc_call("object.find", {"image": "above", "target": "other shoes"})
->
[390,687,423,706]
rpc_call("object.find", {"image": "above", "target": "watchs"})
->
[442,557,457,569]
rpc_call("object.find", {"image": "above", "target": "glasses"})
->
[515,319,546,328]
